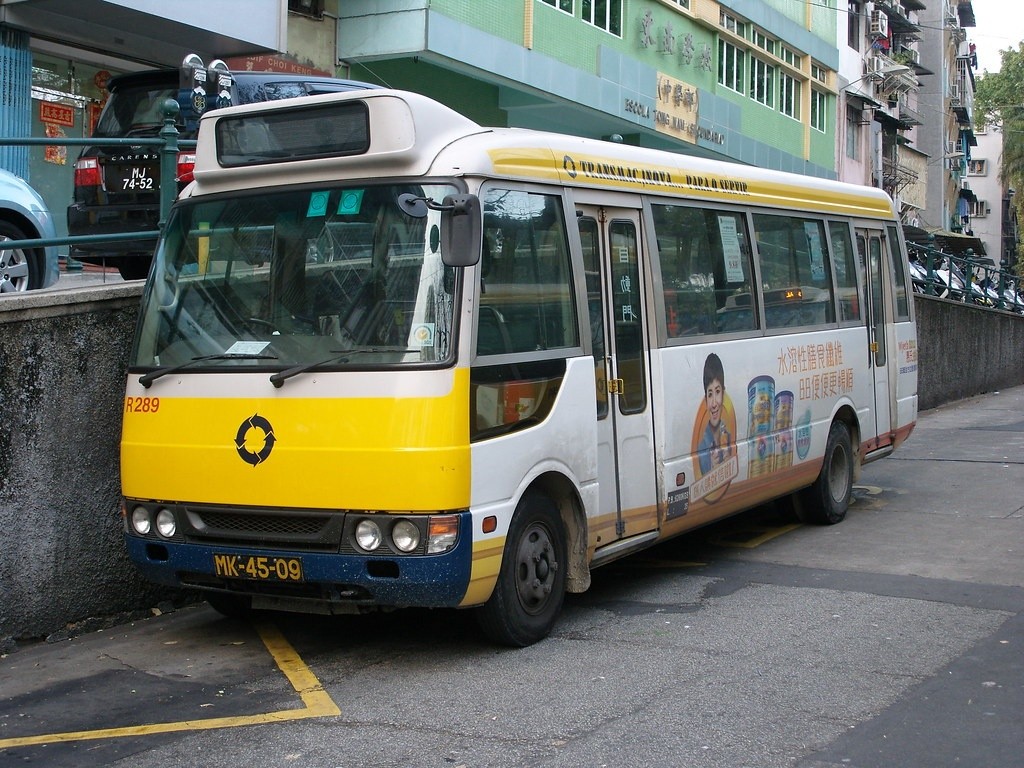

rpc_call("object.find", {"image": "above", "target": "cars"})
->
[1,167,59,295]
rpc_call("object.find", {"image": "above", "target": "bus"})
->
[116,90,921,647]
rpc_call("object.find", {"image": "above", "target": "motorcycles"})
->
[905,248,1024,313]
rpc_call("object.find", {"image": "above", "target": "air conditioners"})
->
[947,141,956,157]
[951,85,961,106]
[953,214,962,227]
[869,10,889,38]
[866,57,884,83]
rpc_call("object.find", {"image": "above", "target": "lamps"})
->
[839,65,909,92]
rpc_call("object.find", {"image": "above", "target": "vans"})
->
[68,65,393,279]
